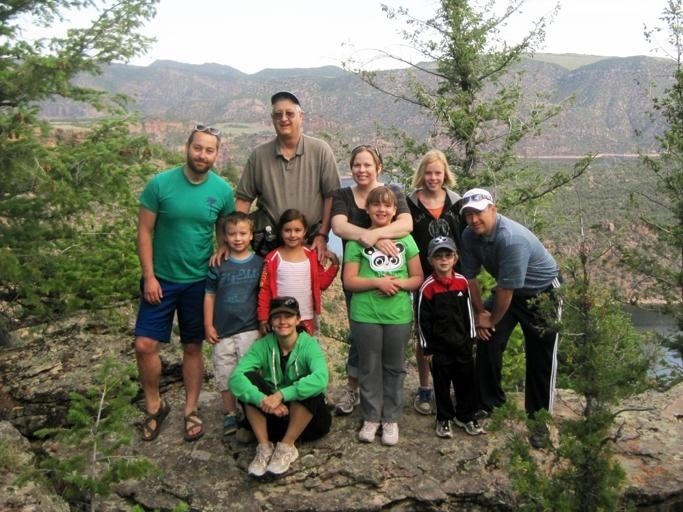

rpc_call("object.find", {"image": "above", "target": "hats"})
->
[267,296,302,318]
[456,187,495,212]
[426,235,458,257]
[270,89,302,107]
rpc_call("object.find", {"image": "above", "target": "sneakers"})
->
[437,421,455,438]
[455,414,486,436]
[413,386,435,417]
[246,442,301,478]
[220,408,239,437]
[526,427,550,449]
[359,420,402,447]
[334,386,362,417]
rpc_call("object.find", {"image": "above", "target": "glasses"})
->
[460,192,485,203]
[195,121,221,138]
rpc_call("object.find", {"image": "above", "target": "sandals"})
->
[185,408,204,443]
[139,402,169,441]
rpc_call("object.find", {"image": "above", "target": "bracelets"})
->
[315,233,329,242]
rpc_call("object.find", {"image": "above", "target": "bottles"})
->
[265,225,278,250]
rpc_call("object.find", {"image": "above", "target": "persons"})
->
[133,124,237,442]
[405,149,468,414]
[227,296,331,478]
[342,186,425,446]
[330,145,414,415]
[208,91,341,268]
[416,235,482,440]
[459,188,565,449]
[257,209,340,336]
[203,211,264,436]
